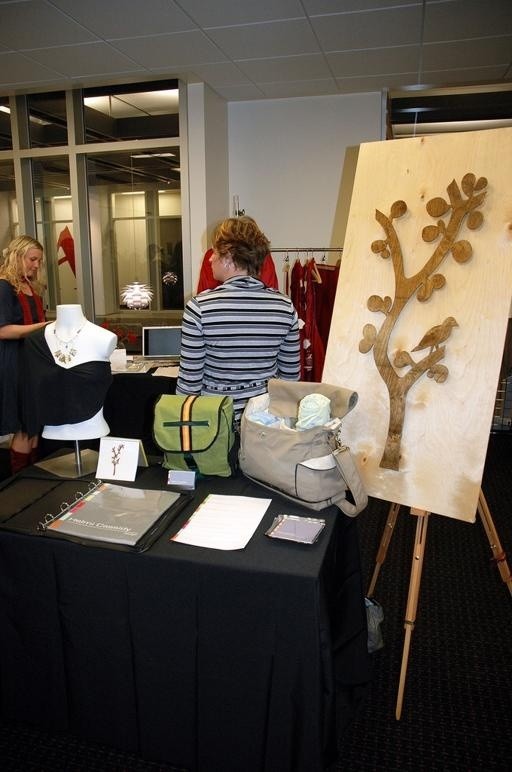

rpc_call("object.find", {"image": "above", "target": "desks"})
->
[103,356,179,428]
[0,448,357,765]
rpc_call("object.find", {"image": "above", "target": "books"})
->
[1,470,194,553]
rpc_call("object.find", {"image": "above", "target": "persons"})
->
[1,235,54,475]
[20,304,119,441]
[175,218,301,447]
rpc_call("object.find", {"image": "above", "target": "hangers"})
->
[280,250,335,286]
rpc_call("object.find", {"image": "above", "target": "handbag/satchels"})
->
[238,379,369,520]
[153,393,236,478]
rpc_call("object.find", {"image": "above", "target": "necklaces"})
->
[52,319,86,364]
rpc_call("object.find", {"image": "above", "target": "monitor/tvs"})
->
[142,326,182,359]
